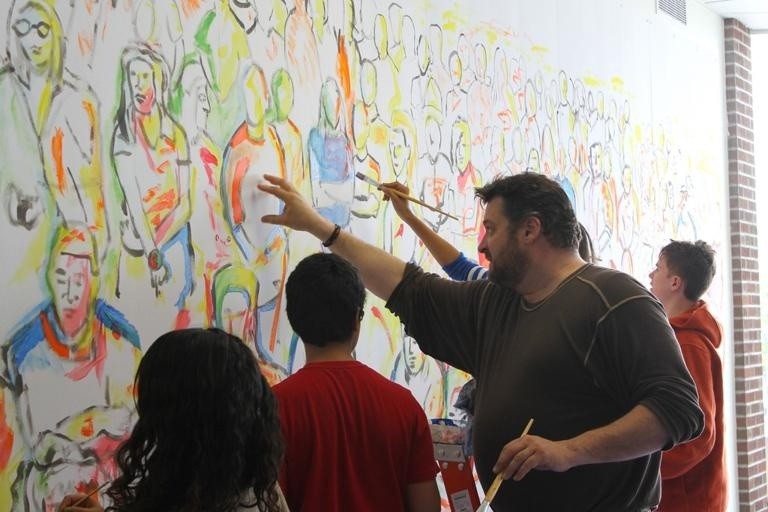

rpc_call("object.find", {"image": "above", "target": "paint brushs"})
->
[475,418,534,512]
[355,171,459,221]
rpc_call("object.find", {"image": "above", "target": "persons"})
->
[106,329,291,510]
[646,239,729,512]
[377,182,595,282]
[259,172,702,512]
[272,253,443,512]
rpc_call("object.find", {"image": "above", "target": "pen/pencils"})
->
[72,481,110,506]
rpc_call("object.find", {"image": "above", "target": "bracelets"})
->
[324,223,341,246]
